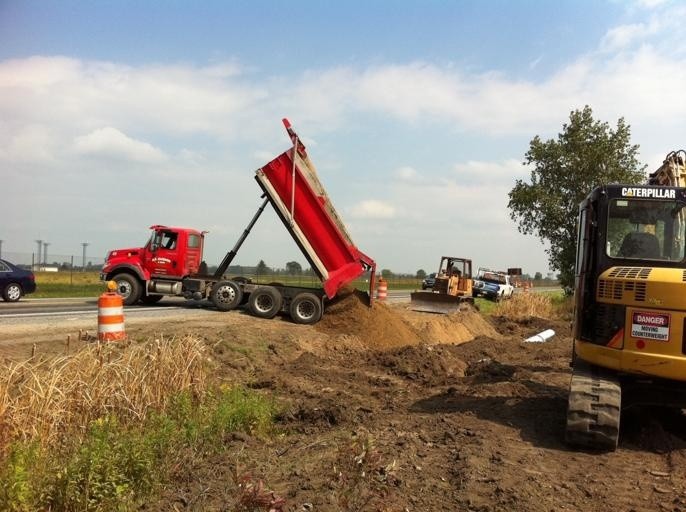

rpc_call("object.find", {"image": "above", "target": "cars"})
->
[0,258,35,301]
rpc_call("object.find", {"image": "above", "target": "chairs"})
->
[614,230,662,259]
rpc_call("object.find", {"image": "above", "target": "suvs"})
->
[420,273,438,291]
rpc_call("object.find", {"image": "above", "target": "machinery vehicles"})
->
[409,256,474,314]
[561,148,686,454]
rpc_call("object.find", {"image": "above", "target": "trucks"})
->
[473,271,514,302]
[97,117,377,327]
